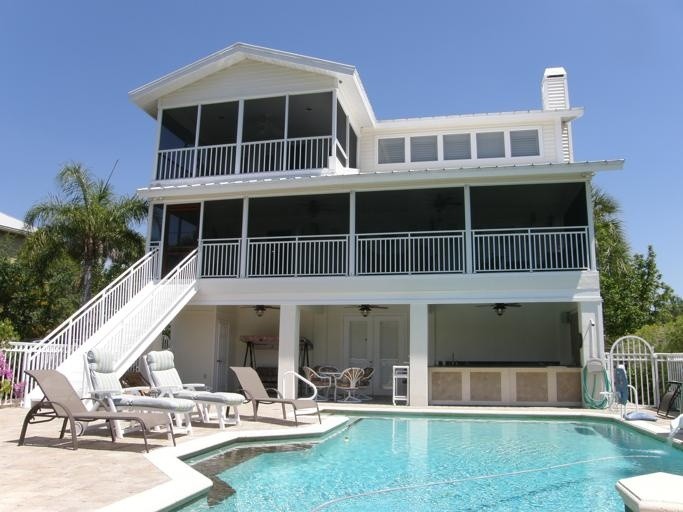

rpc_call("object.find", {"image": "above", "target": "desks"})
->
[321,372,342,401]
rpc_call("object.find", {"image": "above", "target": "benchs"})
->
[239,336,314,395]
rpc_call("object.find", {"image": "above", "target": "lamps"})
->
[256,309,264,317]
[495,308,504,315]
[362,309,368,317]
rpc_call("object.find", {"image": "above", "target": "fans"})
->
[239,305,280,317]
[345,305,388,318]
[476,304,522,317]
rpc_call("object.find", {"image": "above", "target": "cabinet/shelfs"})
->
[392,366,409,405]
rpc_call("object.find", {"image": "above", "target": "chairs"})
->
[16,369,176,453]
[228,365,322,427]
[318,365,342,400]
[353,366,376,401]
[142,349,245,431]
[302,366,332,402]
[336,367,366,403]
[83,349,196,441]
[614,364,656,422]
